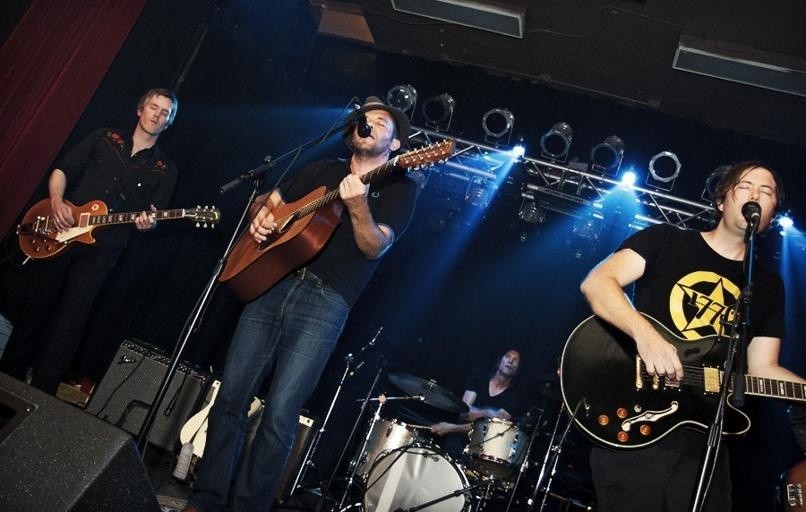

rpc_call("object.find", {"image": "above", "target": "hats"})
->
[341,95,409,149]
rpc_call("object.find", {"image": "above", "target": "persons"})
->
[431,342,539,465]
[180,95,419,511]
[579,158,806,511]
[2,87,180,395]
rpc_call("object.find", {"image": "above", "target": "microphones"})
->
[353,97,372,140]
[740,201,763,230]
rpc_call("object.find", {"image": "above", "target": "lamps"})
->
[540,121,574,157]
[461,186,493,229]
[407,169,427,197]
[510,199,549,244]
[648,143,683,182]
[590,135,625,171]
[387,84,416,111]
[566,219,598,261]
[482,107,514,138]
[421,92,455,125]
[699,165,733,202]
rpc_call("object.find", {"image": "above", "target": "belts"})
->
[293,267,329,284]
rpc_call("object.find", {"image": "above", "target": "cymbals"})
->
[387,370,470,416]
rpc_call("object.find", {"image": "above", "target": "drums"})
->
[354,419,417,479]
[362,442,473,512]
[461,416,532,473]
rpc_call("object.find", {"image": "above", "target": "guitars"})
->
[218,138,457,305]
[15,197,221,265]
[559,309,806,454]
